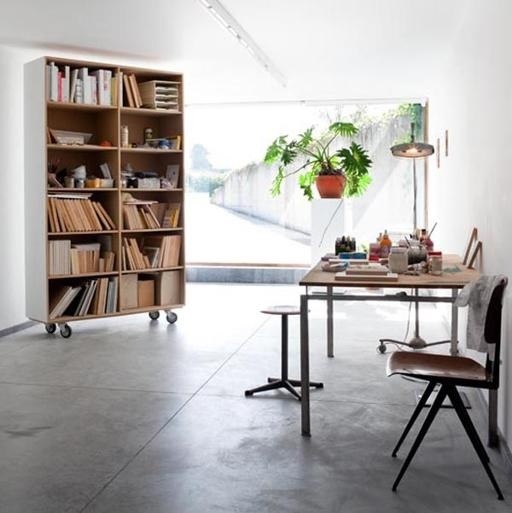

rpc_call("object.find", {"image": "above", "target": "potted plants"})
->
[261,117,373,203]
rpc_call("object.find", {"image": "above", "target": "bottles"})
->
[120,124,128,147]
[63,177,84,188]
[388,247,408,274]
[379,229,392,258]
[427,251,443,275]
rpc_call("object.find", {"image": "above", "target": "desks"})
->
[297,252,485,440]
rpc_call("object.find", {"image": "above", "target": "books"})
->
[329,258,398,281]
[45,61,182,319]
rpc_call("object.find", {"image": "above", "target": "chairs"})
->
[381,275,509,503]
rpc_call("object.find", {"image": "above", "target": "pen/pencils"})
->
[335,236,356,249]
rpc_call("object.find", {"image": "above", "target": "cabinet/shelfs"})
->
[21,55,187,340]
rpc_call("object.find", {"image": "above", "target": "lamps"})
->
[378,122,461,355]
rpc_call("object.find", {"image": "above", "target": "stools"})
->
[244,304,324,402]
